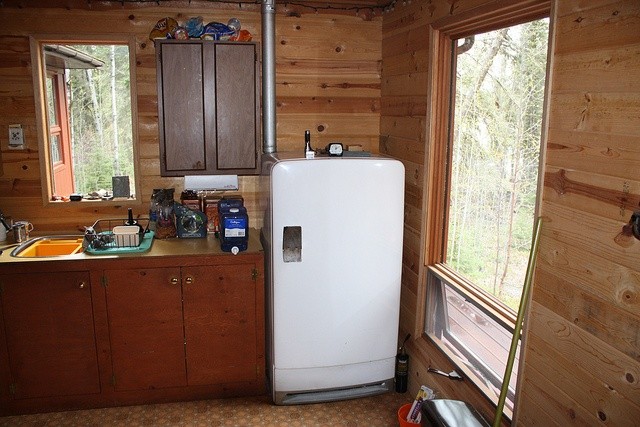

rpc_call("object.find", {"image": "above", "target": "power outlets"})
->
[9,128,23,145]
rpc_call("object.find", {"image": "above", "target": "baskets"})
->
[85,216,150,247]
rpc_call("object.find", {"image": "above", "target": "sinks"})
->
[10,235,84,258]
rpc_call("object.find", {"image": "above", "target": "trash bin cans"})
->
[398,404,426,427]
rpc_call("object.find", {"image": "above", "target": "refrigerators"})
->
[270,156,406,405]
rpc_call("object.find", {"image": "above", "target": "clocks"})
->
[328,143,343,156]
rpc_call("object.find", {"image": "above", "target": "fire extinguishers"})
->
[396,334,411,393]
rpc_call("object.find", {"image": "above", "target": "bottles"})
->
[13,222,26,242]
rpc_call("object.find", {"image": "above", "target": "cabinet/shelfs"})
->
[155,40,261,177]
[0,262,103,413]
[101,255,264,407]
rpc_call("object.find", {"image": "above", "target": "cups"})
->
[16,221,34,241]
